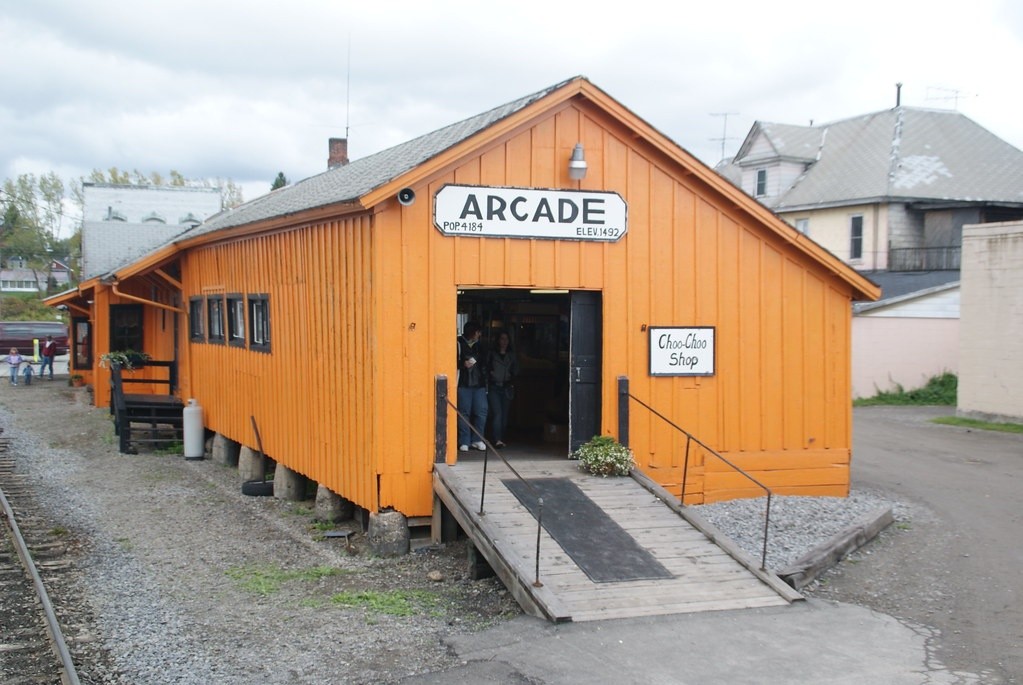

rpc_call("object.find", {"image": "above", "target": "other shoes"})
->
[48,377,53,380]
[496,440,506,448]
[14,383,17,386]
[25,382,31,385]
[35,376,43,379]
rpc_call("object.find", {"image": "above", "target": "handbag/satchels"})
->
[506,382,515,399]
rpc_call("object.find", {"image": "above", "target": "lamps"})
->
[569,142,589,179]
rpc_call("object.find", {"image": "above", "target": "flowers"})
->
[571,435,642,478]
[98,350,153,372]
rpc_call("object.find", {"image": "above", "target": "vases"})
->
[597,464,614,476]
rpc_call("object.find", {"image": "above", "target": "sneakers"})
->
[460,444,469,452]
[472,441,486,450]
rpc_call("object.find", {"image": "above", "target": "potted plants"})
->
[67,374,83,387]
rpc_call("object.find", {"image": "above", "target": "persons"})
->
[22,361,35,385]
[37,334,56,380]
[7,347,23,386]
[457,320,488,452]
[485,329,518,449]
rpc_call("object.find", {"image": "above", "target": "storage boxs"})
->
[544,423,570,441]
